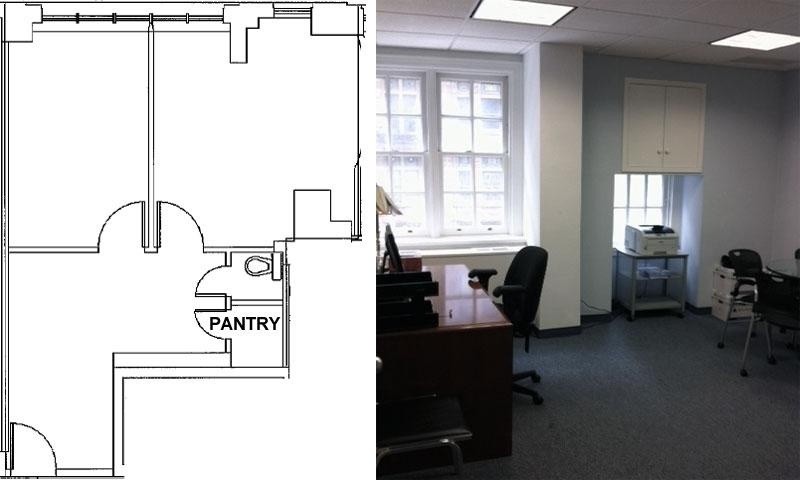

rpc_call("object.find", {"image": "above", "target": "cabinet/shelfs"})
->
[623,76,708,173]
[614,246,689,324]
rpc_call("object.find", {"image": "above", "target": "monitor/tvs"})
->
[383,221,406,273]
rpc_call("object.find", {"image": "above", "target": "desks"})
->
[377,262,513,471]
[767,260,799,281]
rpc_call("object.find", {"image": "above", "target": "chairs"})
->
[467,246,548,405]
[721,248,799,377]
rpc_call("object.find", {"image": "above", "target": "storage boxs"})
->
[711,263,762,320]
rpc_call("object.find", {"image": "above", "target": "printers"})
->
[623,221,681,258]
